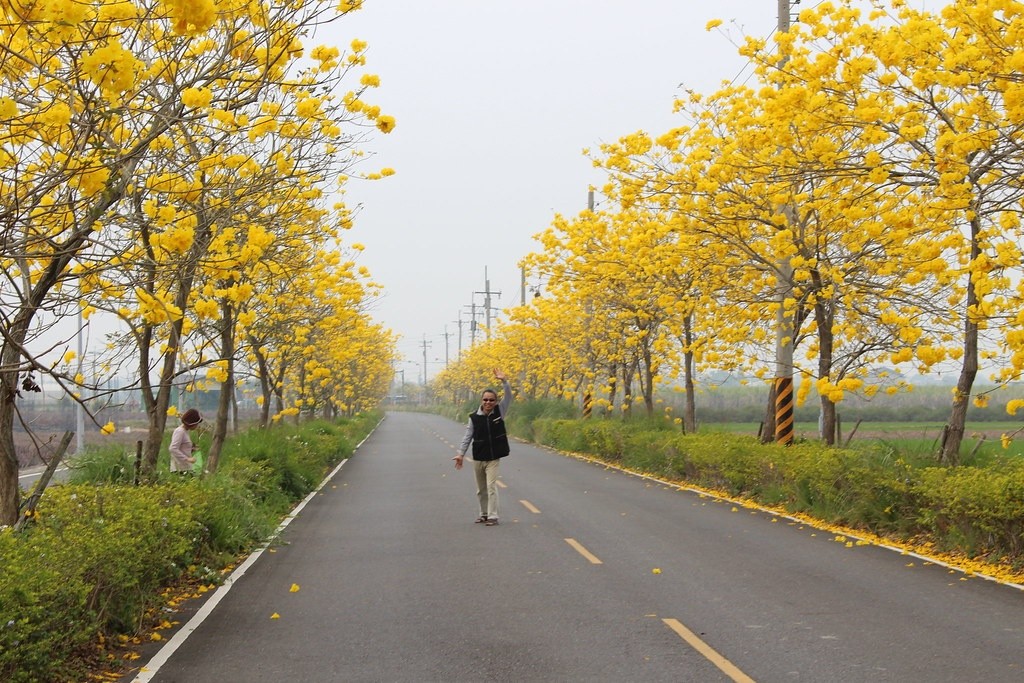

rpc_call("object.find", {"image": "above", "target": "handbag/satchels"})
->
[191,451,203,477]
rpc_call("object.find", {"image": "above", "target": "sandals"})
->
[486,519,497,525]
[475,516,488,523]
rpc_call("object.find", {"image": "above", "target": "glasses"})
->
[482,398,496,402]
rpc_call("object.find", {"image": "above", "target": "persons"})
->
[452,367,511,526]
[169,409,202,476]
